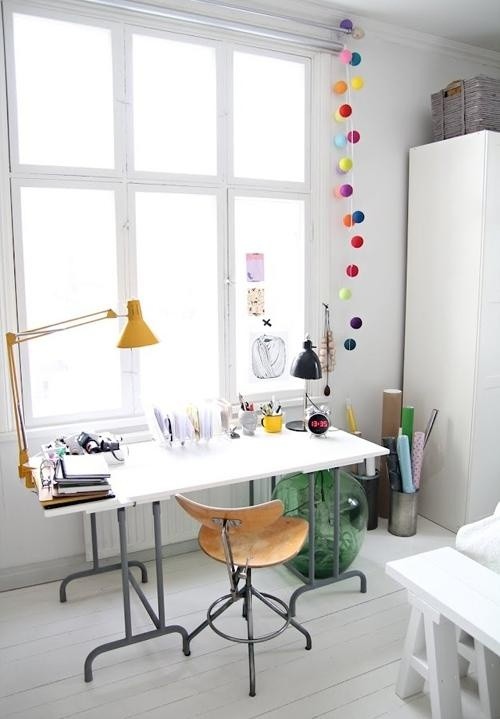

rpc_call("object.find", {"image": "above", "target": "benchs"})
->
[385,547,499,718]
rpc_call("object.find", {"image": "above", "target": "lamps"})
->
[287,338,322,432]
[5,299,159,489]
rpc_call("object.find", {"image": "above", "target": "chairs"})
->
[174,493,313,700]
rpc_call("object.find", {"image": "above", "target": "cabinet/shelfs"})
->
[400,126,500,530]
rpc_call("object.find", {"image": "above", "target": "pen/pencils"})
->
[238,393,256,412]
[260,400,283,417]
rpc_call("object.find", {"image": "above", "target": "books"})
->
[38,455,116,508]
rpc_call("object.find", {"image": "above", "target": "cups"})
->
[237,409,258,436]
[262,413,284,434]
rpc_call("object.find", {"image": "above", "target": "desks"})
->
[36,417,395,682]
